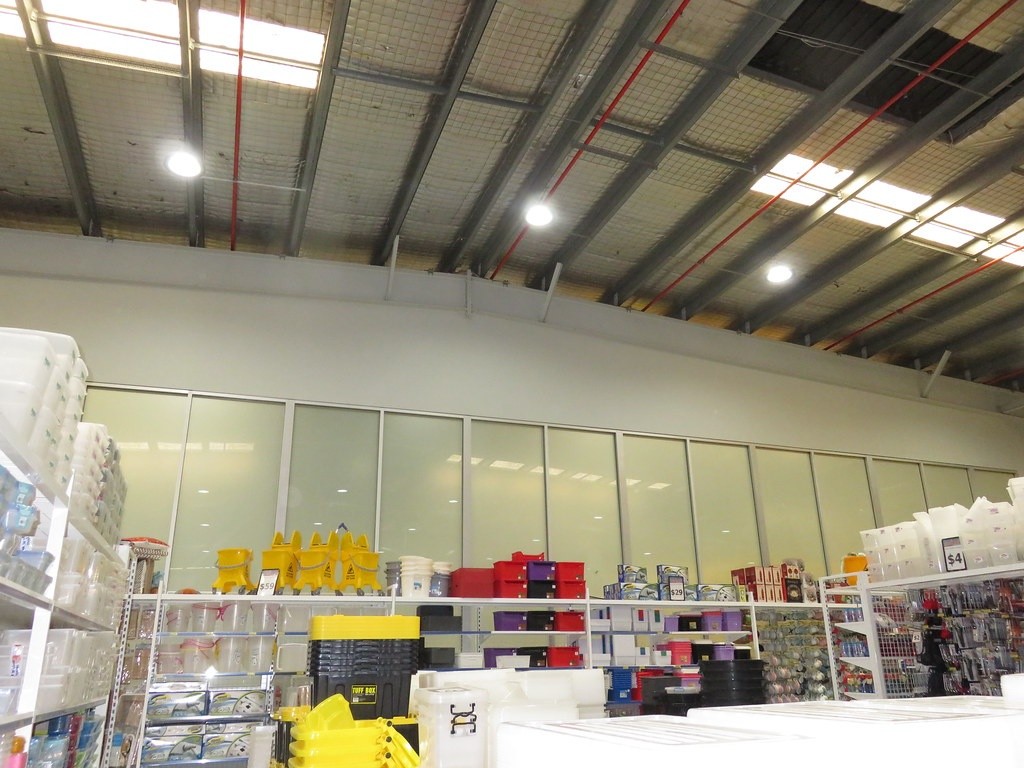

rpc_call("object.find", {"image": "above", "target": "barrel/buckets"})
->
[700,659,770,705]
[384,556,452,597]
[642,676,701,716]
[158,604,390,672]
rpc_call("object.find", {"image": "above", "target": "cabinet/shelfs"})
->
[0,414,137,768]
[124,580,862,768]
[826,561,1024,700]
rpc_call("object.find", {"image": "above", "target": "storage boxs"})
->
[384,556,451,597]
[527,560,556,580]
[483,646,580,668]
[141,691,266,764]
[493,611,527,630]
[604,564,818,717]
[555,562,585,579]
[305,605,482,721]
[0,327,131,768]
[860,476,1024,583]
[525,611,556,631]
[554,612,584,631]
[494,580,528,598]
[556,580,586,599]
[448,567,494,598]
[512,551,544,562]
[527,580,556,598]
[493,561,527,580]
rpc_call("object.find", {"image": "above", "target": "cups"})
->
[26,712,104,768]
